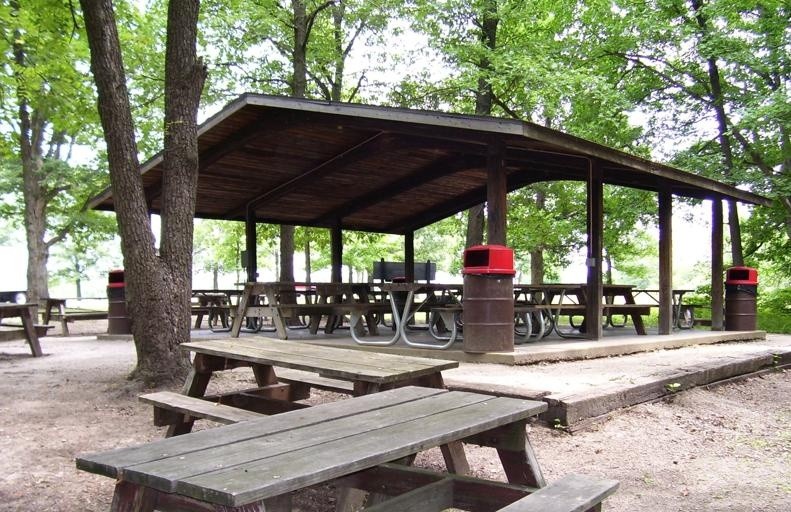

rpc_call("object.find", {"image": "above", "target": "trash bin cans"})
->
[723,267,759,330]
[107,271,128,334]
[461,245,514,353]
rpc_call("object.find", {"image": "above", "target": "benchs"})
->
[138,379,275,433]
[190,299,438,338]
[5,321,56,338]
[335,299,651,343]
[673,304,703,330]
[423,470,623,512]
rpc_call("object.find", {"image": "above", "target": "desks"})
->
[197,281,462,339]
[0,303,44,357]
[630,289,695,330]
[164,334,460,438]
[74,383,549,512]
[383,280,638,348]
